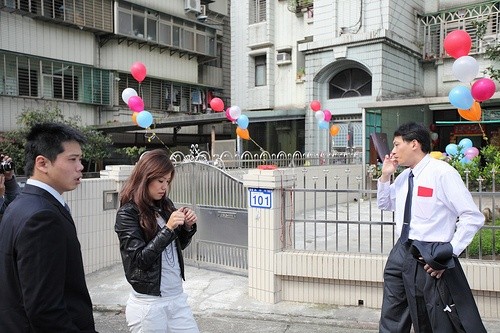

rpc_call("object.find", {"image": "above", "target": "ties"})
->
[399,171,413,245]
[64,203,72,215]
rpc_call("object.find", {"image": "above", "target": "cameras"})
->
[0,156,16,174]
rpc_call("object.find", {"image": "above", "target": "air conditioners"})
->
[183,0,206,16]
[277,53,290,62]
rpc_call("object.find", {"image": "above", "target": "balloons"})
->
[210,98,250,140]
[443,30,496,121]
[130,61,147,83]
[121,88,153,128]
[311,100,339,136]
[445,138,478,164]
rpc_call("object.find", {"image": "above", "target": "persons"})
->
[377,123,486,333]
[114,149,200,333]
[0,154,21,214]
[0,122,100,333]
